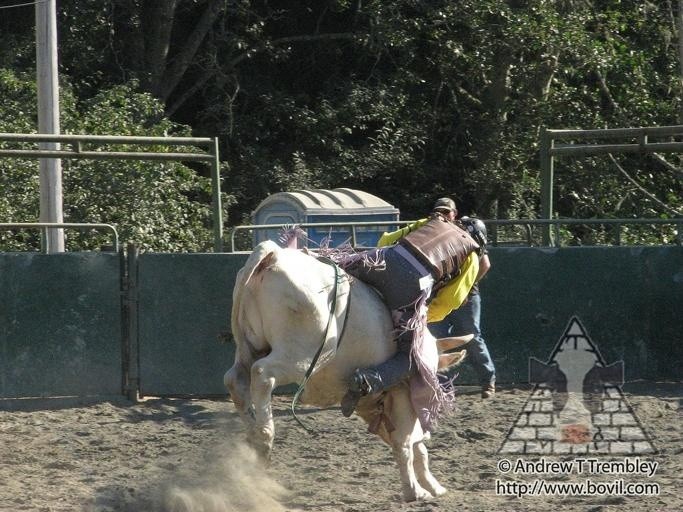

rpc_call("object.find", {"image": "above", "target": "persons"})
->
[342,209,489,418]
[427,198,497,397]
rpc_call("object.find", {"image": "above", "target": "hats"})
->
[434,197,455,211]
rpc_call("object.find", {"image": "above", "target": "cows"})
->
[222,239,476,505]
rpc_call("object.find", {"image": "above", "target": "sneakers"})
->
[481,382,496,397]
[341,373,364,417]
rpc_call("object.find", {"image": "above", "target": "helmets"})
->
[461,215,487,248]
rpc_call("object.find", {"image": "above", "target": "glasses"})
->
[435,209,450,215]
